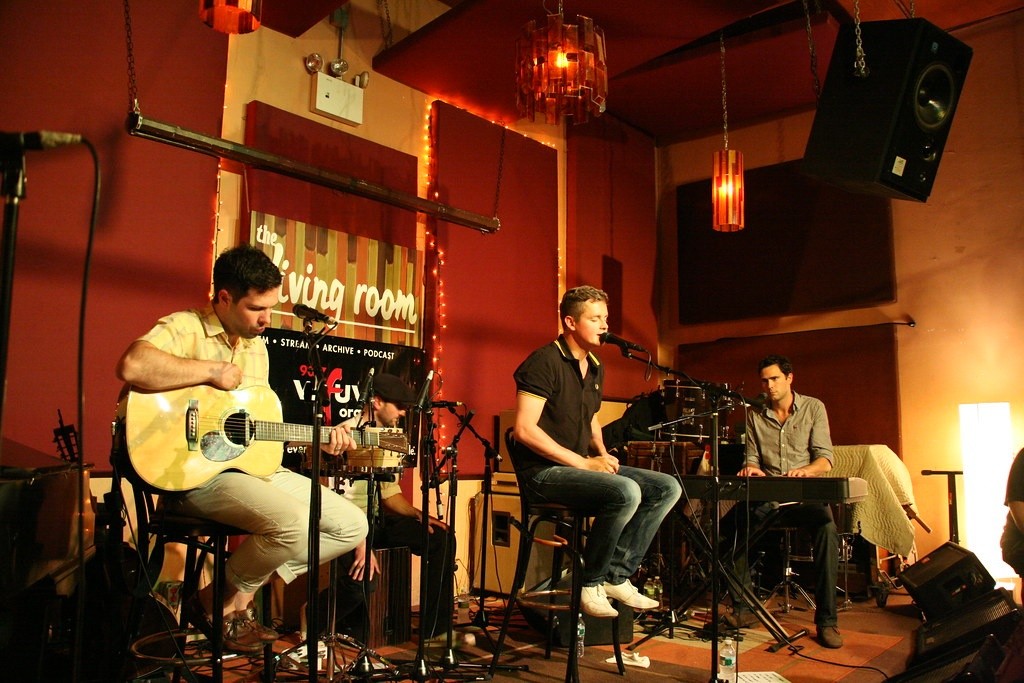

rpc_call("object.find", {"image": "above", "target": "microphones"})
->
[432,401,463,408]
[419,470,458,490]
[600,332,647,352]
[292,303,339,325]
[413,370,434,414]
[356,367,374,409]
[0,130,84,158]
[752,392,768,403]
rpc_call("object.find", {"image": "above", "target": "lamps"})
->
[306,54,369,126]
[198,0,263,35]
[713,36,745,232]
[514,0,609,125]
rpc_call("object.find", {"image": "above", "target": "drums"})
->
[661,378,730,440]
[336,424,405,474]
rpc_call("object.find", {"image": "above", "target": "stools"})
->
[768,519,818,615]
[118,418,275,683]
[481,428,627,683]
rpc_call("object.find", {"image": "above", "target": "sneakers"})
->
[604,579,659,608]
[580,584,618,617]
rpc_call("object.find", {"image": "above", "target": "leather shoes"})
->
[189,590,263,654]
[234,601,278,640]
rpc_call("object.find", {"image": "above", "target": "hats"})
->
[373,373,415,402]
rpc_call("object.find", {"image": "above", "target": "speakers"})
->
[898,540,997,621]
[881,633,1006,683]
[916,587,1023,664]
[802,17,972,204]
[521,571,634,647]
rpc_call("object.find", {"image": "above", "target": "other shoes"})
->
[817,625,844,648]
[721,612,758,630]
[425,631,465,646]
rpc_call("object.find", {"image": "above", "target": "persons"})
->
[115,245,369,659]
[702,356,846,651]
[297,372,475,653]
[998,448,1023,583]
[510,285,682,617]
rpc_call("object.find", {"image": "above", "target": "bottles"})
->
[718,639,736,683]
[577,613,585,658]
[654,576,664,610]
[458,588,469,623]
[643,578,655,610]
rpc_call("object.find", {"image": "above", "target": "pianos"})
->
[625,473,870,653]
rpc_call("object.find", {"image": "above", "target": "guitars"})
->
[51,421,181,680]
[116,380,413,495]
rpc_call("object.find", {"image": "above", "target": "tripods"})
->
[258,327,528,683]
[626,402,749,651]
